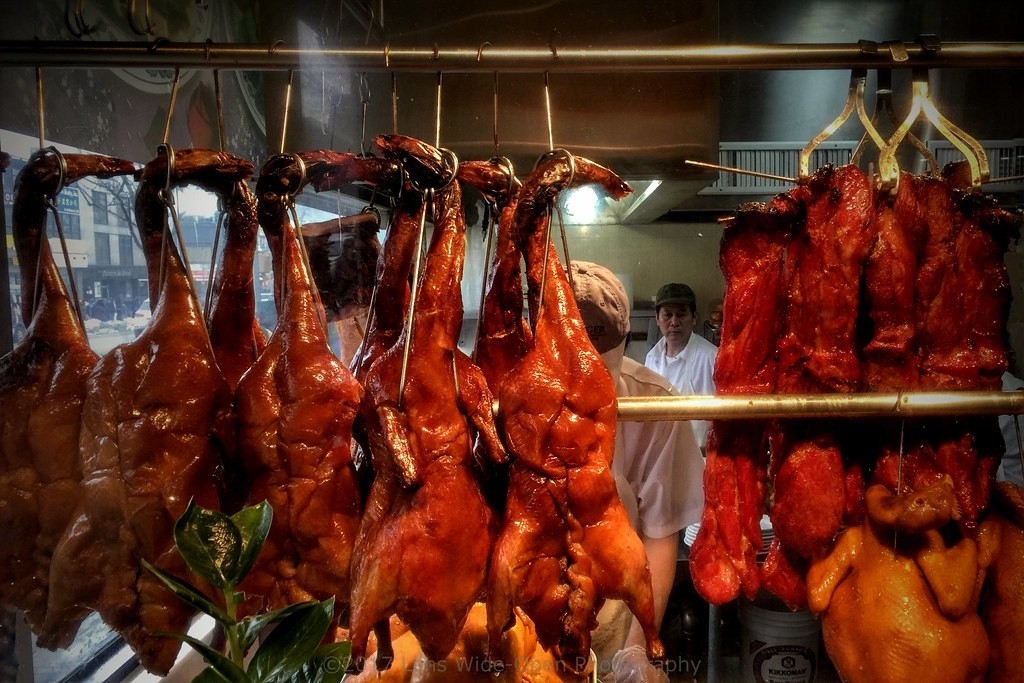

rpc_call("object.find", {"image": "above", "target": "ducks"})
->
[0,134,667,672]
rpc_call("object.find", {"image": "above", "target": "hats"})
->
[652,284,697,309]
[561,260,630,354]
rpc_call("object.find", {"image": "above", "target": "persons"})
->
[558,261,707,683]
[642,282,720,456]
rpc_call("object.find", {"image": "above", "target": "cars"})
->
[133,297,151,336]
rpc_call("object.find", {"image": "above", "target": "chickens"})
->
[808,477,1024,683]
